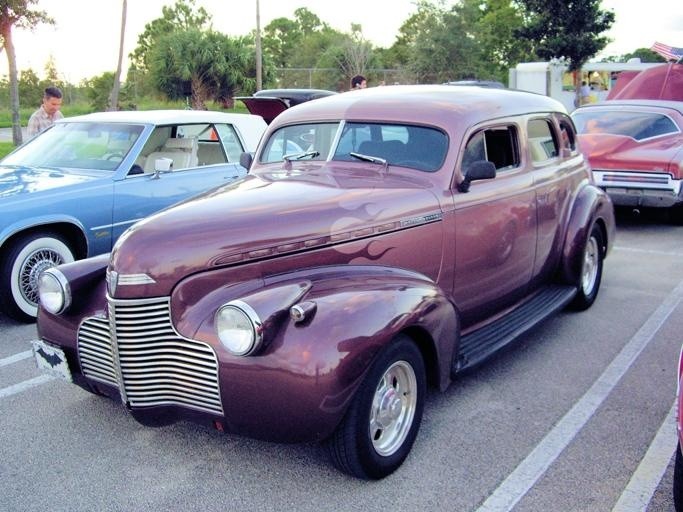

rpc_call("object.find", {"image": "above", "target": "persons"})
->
[350,75,368,91]
[26,86,65,139]
[580,82,592,99]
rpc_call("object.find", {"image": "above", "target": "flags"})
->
[649,40,682,62]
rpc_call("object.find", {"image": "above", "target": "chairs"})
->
[99,136,198,174]
[356,139,404,166]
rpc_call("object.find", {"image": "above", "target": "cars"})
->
[566,57,682,223]
[1,108,279,326]
[29,83,617,483]
